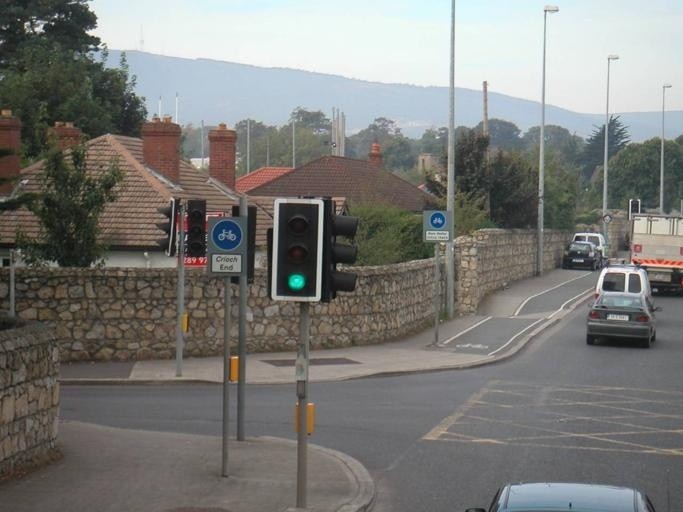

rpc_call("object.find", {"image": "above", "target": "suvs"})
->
[594,260,656,312]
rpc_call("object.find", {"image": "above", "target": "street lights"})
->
[660,83,672,213]
[602,54,619,258]
[536,4,559,277]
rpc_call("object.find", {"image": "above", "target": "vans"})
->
[572,232,609,267]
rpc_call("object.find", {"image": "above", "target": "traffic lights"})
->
[271,194,359,303]
[154,196,206,257]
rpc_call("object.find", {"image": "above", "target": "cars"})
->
[585,291,663,348]
[562,241,601,271]
[465,482,656,512]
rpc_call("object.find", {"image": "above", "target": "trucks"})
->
[624,213,683,297]
